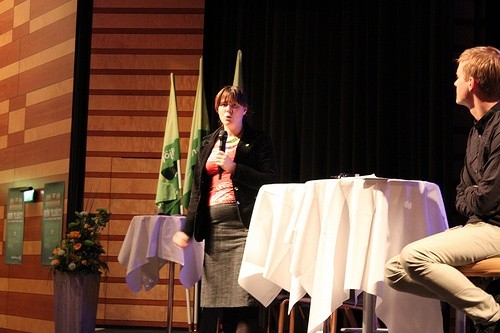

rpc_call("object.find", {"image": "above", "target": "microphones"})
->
[217,131,227,179]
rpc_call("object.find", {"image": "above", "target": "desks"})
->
[117,215,209,333]
[238,178,450,333]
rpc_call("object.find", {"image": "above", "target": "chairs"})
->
[278,289,363,333]
[442,257,500,333]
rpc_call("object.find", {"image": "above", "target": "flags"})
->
[156,85,180,215]
[182,74,209,209]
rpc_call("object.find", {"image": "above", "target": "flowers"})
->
[49,207,111,277]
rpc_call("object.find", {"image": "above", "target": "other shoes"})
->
[476,319,500,333]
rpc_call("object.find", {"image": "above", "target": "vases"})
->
[54,272,102,333]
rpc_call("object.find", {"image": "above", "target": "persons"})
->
[384,46,500,333]
[173,86,279,333]
